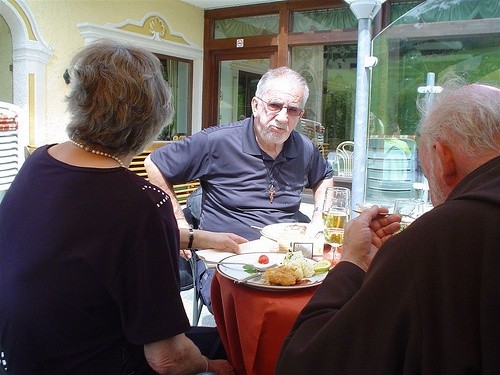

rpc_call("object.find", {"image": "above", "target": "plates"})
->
[260,222,308,244]
[215,251,330,292]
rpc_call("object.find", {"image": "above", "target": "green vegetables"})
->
[242,264,259,274]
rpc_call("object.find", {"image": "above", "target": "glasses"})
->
[256,96,301,117]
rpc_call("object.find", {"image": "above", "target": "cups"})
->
[393,199,426,235]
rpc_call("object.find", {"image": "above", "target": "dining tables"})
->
[210,222,341,375]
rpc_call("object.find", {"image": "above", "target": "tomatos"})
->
[258,255,269,264]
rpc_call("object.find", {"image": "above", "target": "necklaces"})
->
[264,161,283,203]
[69,139,127,168]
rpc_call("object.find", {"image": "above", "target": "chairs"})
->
[186,185,205,326]
[336,141,356,177]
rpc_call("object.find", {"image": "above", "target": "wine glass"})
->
[322,207,348,271]
[322,186,351,260]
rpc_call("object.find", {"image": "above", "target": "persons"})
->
[143,65,335,316]
[0,37,234,375]
[273,81,500,375]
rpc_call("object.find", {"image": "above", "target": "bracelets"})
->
[187,228,194,251]
[201,355,209,373]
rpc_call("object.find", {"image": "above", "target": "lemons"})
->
[314,260,331,272]
